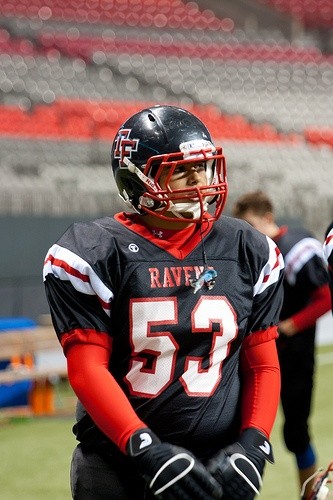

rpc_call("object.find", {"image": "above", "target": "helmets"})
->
[111,104,227,223]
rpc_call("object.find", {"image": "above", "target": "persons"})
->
[323,221,333,315]
[43,105,286,500]
[235,194,330,500]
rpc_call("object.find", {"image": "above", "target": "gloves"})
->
[126,428,223,500]
[206,428,274,500]
